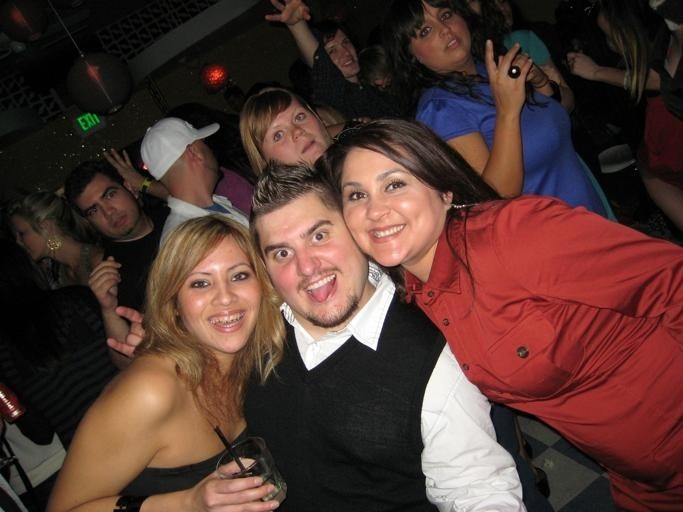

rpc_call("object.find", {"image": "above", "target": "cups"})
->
[216,436,287,506]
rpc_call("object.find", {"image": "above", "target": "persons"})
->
[106,165,526,511]
[48,213,286,512]
[328,116,681,510]
[381,2,681,230]
[0,1,412,486]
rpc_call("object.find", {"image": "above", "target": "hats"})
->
[141,117,220,181]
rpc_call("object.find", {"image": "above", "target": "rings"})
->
[507,66,521,79]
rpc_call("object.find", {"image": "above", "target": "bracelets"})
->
[532,75,544,84]
[140,178,154,193]
[623,70,630,90]
[536,76,549,90]
[550,81,562,104]
